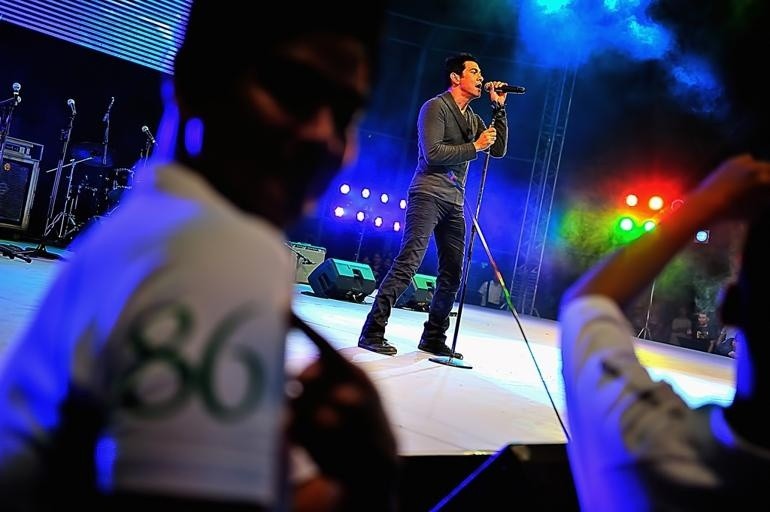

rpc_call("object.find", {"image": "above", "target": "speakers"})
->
[307,257,376,303]
[393,273,438,307]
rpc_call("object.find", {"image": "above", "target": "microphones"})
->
[142,126,156,145]
[12,82,21,104]
[68,99,77,115]
[485,82,525,96]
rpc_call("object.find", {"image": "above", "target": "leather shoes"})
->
[357,332,397,356]
[418,337,464,360]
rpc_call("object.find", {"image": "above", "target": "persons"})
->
[558,154,768,510]
[479,270,507,309]
[383,255,394,272]
[370,253,385,286]
[357,52,510,359]
[667,307,739,359]
[1,0,381,512]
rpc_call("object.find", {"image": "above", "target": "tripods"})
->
[636,279,656,340]
[0,106,32,263]
[10,116,75,263]
[44,162,80,241]
